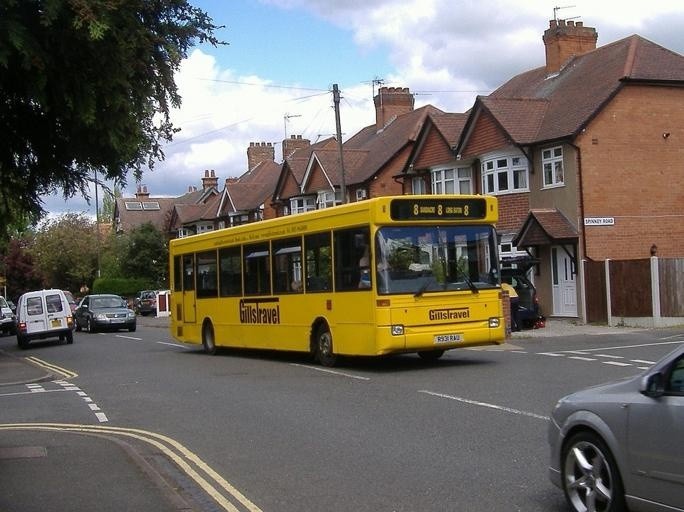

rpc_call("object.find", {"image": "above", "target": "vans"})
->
[16,288,73,347]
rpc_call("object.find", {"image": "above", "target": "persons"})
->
[501,278,522,332]
[358,234,390,289]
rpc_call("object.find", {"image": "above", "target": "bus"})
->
[168,194,505,365]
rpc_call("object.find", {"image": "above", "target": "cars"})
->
[0,295,17,336]
[134,290,158,314]
[64,291,135,333]
[501,257,542,331]
[549,344,684,512]
